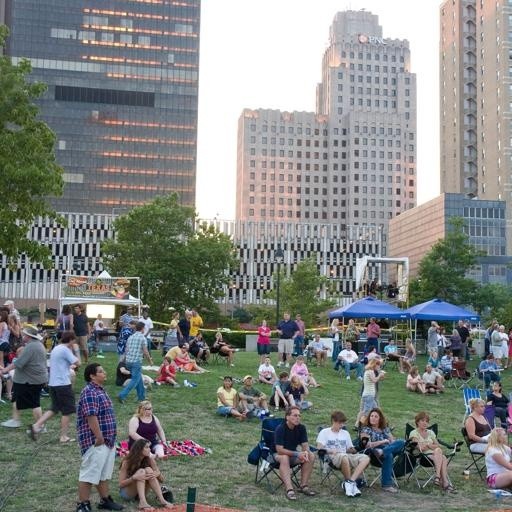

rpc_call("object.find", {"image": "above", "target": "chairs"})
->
[405,423,464,490]
[211,339,228,366]
[462,388,482,425]
[401,349,414,371]
[337,348,357,384]
[486,390,510,429]
[189,337,206,366]
[358,341,366,361]
[307,341,328,365]
[254,419,317,493]
[507,403,512,446]
[380,342,399,371]
[449,360,476,389]
[352,421,421,488]
[467,347,478,360]
[477,366,501,392]
[318,427,367,493]
[461,427,487,481]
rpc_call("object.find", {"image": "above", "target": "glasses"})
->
[225,375,232,378]
[146,408,151,410]
[291,414,302,417]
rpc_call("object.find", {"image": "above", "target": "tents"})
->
[329,296,412,364]
[403,298,482,356]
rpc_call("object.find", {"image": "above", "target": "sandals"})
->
[443,484,458,494]
[287,489,297,500]
[297,484,316,496]
[433,477,441,486]
[352,425,358,431]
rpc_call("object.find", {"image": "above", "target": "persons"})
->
[426,318,474,362]
[364,317,383,356]
[409,412,458,494]
[238,375,270,417]
[330,318,345,363]
[91,313,109,350]
[294,312,305,357]
[118,439,174,512]
[73,304,91,368]
[485,428,512,489]
[269,406,316,500]
[441,350,459,380]
[352,358,395,432]
[364,346,382,362]
[365,278,399,299]
[422,364,445,394]
[483,319,512,370]
[256,320,272,367]
[406,366,428,395]
[337,341,364,382]
[285,375,316,408]
[29,331,81,444]
[54,304,72,343]
[153,356,180,389]
[316,411,370,498]
[402,338,416,374]
[0,300,48,435]
[384,338,404,375]
[258,357,277,386]
[116,303,154,404]
[486,381,510,432]
[75,363,124,512]
[165,342,188,362]
[290,356,322,388]
[188,332,213,365]
[163,309,203,340]
[128,401,167,461]
[465,398,492,454]
[210,331,241,368]
[269,371,291,412]
[308,335,331,368]
[174,342,210,375]
[345,320,361,356]
[479,355,505,390]
[360,408,407,494]
[275,311,302,368]
[427,350,443,378]
[217,376,246,423]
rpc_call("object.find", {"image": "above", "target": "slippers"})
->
[29,423,39,443]
[59,437,77,444]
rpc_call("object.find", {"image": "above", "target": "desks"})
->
[97,332,118,343]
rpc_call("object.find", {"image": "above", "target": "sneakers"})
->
[354,481,362,496]
[381,486,399,493]
[75,500,93,512]
[97,495,124,511]
[341,479,354,498]
[1,419,21,428]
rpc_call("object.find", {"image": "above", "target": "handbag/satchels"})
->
[156,486,174,502]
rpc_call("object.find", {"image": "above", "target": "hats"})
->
[3,300,14,306]
[242,374,252,382]
[22,325,40,338]
